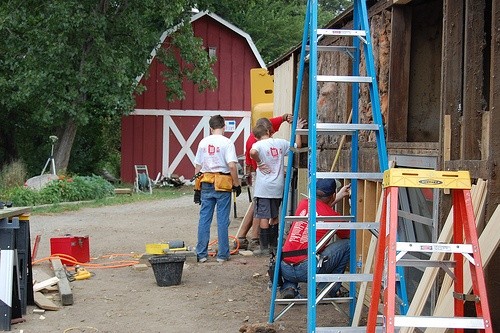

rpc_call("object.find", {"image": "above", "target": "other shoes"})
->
[277,288,296,306]
[316,288,341,300]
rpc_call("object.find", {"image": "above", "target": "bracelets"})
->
[256,160,262,164]
[285,114,289,121]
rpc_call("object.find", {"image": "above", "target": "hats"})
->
[316,178,337,198]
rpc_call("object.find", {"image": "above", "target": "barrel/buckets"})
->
[149,255,187,286]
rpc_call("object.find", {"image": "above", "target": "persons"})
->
[193,115,242,263]
[268,178,353,304]
[236,112,296,247]
[249,116,308,253]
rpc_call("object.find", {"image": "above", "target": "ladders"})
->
[364,168,492,333]
[265,1,408,333]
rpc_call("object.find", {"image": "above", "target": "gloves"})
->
[232,184,241,197]
[193,188,202,206]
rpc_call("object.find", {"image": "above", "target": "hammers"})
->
[241,174,252,202]
[169,245,191,251]
[317,255,333,274]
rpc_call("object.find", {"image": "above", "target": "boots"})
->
[258,222,279,256]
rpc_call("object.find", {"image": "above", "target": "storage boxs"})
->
[148,254,187,287]
[50,234,92,266]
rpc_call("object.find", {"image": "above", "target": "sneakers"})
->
[233,236,261,251]
